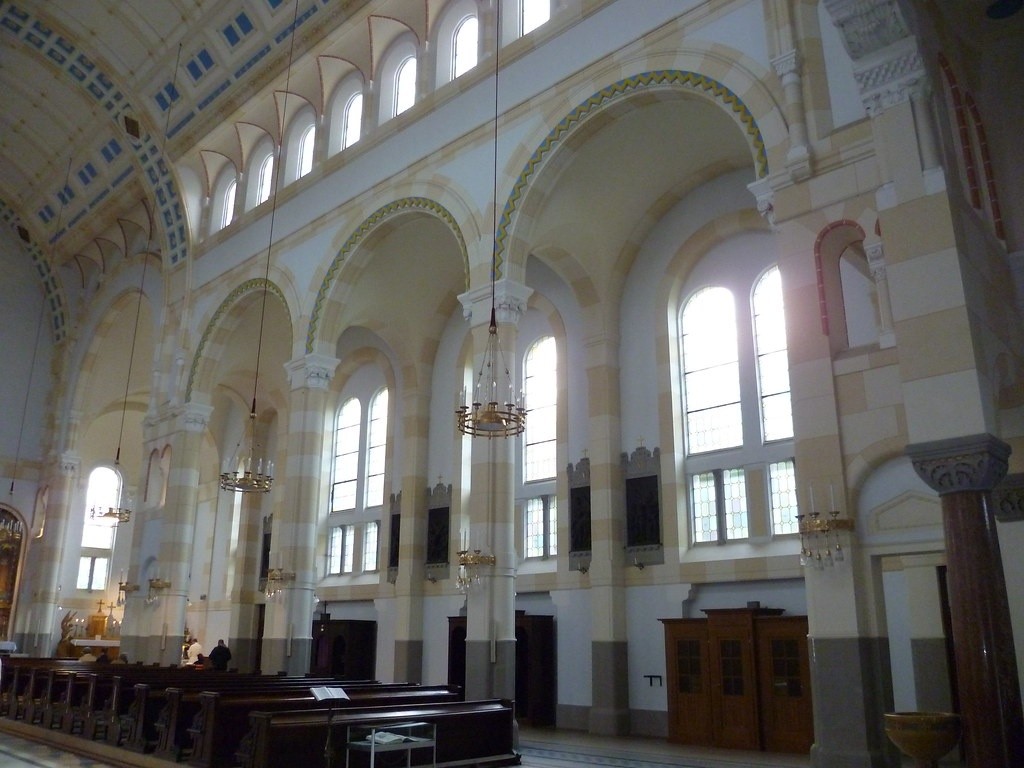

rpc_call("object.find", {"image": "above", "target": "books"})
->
[367,732,430,745]
[310,686,350,700]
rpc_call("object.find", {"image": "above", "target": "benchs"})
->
[1,655,522,768]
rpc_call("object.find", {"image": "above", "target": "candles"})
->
[476,530,479,550]
[830,484,834,511]
[810,486,815,513]
[464,531,466,551]
[120,571,122,583]
[279,552,282,569]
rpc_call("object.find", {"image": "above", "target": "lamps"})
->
[0,157,72,541]
[455,0,528,440]
[220,0,298,496]
[90,42,183,522]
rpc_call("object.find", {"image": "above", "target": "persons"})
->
[78,647,97,661]
[207,639,231,672]
[60,624,74,639]
[187,639,202,664]
[96,648,112,663]
[193,653,203,664]
[111,652,128,664]
